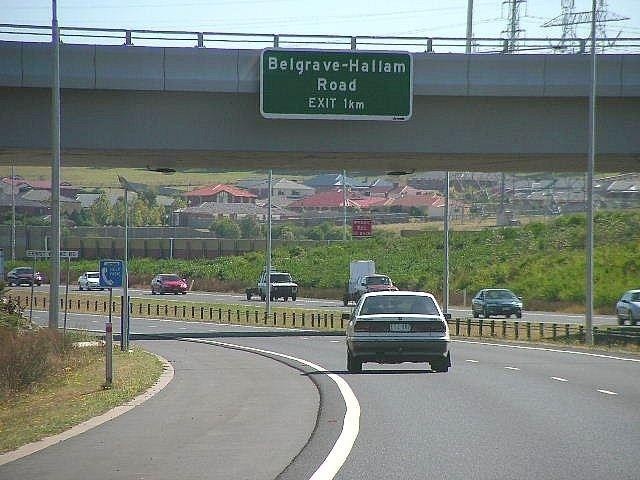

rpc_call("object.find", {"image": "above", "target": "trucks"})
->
[243,264,301,304]
[341,275,403,305]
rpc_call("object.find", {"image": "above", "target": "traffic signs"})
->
[352,218,373,237]
[260,46,412,121]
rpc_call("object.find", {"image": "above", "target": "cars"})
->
[7,267,44,286]
[76,271,108,291]
[616,288,640,325]
[149,273,190,296]
[470,287,525,320]
[341,290,454,374]
